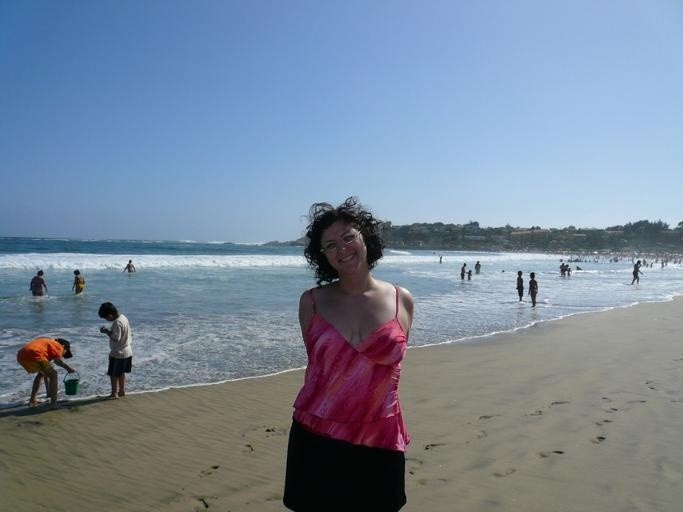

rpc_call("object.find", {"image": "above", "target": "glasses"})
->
[320,230,361,252]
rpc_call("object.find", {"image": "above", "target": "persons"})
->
[439,251,683,309]
[123,260,137,273]
[283,194,414,512]
[98,302,132,399]
[71,270,86,296]
[16,337,76,410]
[29,270,48,297]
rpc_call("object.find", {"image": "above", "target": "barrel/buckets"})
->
[63,371,81,395]
